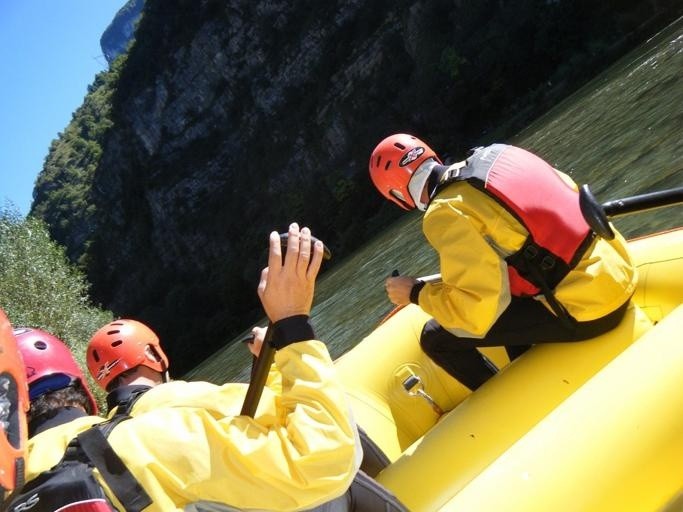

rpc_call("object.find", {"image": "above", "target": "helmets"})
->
[14,327,100,417]
[368,132,446,212]
[0,307,34,512]
[86,318,169,394]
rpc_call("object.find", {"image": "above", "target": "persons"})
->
[368,133,638,392]
[13,222,364,511]
[87,319,282,423]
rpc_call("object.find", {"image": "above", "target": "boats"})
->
[333,222,683,512]
